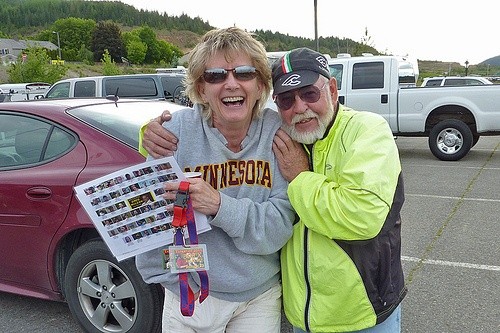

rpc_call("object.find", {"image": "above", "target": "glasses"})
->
[203,66,260,84]
[273,81,329,110]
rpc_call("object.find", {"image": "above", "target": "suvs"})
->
[421,77,494,87]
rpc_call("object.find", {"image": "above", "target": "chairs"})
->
[0,126,57,166]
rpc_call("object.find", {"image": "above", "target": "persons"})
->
[134,27,297,333]
[176,252,205,269]
[81,162,180,245]
[139,47,409,333]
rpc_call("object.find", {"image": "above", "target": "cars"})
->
[0,88,194,333]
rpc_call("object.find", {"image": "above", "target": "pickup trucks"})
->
[324,53,500,160]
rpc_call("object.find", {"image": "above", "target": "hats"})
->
[271,47,332,95]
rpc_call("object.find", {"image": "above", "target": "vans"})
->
[34,71,191,108]
